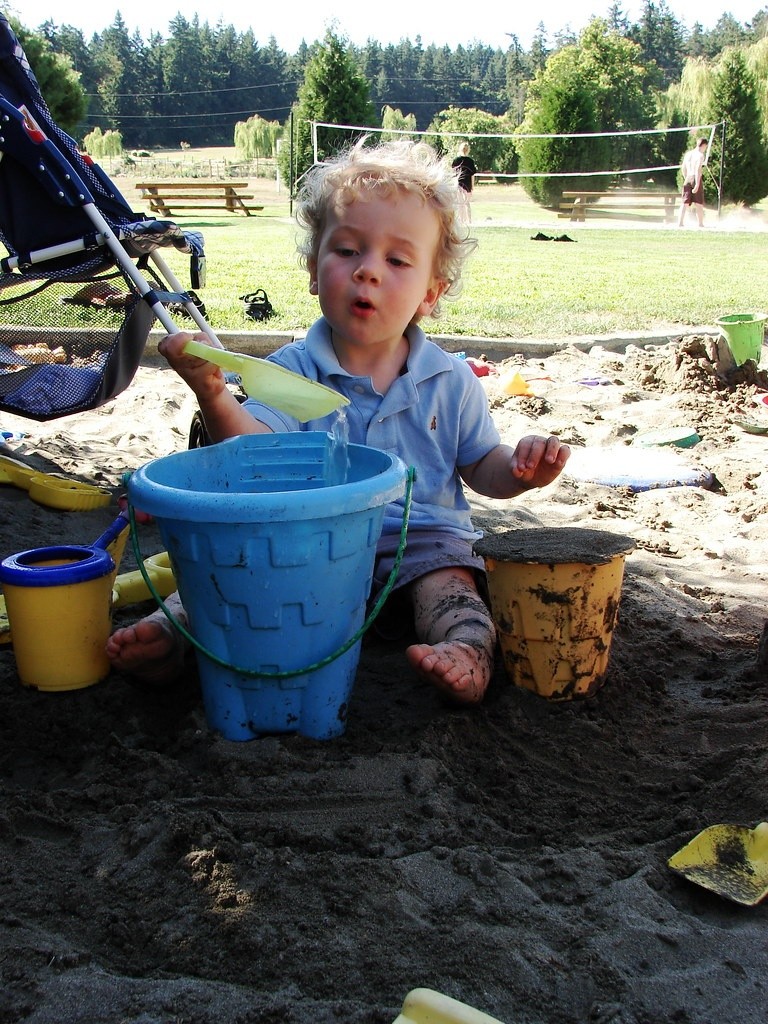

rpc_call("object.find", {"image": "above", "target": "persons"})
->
[103,134,571,700]
[679,138,708,227]
[452,143,477,223]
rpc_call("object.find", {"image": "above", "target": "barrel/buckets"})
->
[124,430,418,744]
[472,526,639,702]
[714,313,768,367]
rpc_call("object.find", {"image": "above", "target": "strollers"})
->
[0,12,249,452]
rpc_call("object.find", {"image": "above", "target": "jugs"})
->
[1,493,152,692]
